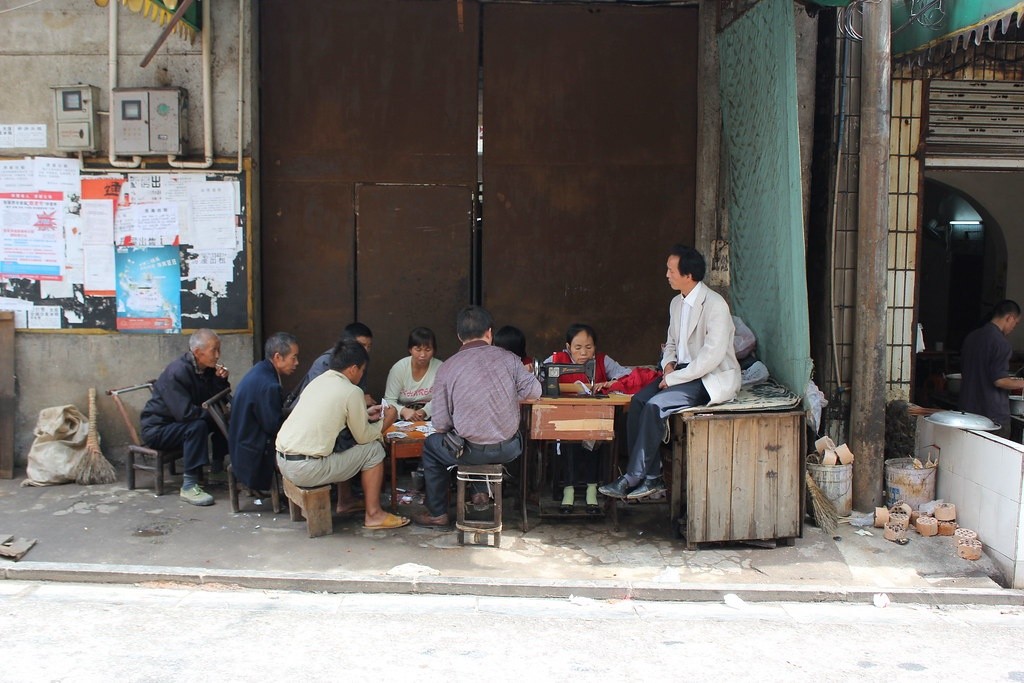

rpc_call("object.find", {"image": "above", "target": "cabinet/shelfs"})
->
[668,381,805,549]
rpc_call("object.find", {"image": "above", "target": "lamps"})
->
[949,220,983,225]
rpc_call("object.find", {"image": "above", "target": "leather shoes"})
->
[627,476,664,498]
[598,476,644,498]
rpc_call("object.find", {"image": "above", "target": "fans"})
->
[921,219,952,240]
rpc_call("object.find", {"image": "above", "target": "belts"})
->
[464,433,517,450]
[276,450,314,460]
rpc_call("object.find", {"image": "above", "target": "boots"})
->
[561,485,574,506]
[586,483,598,505]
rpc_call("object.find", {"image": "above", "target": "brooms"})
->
[66,387,118,485]
[805,469,838,535]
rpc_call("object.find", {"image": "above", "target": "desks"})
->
[376,421,451,514]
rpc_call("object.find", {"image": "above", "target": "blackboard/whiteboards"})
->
[0,155,254,336]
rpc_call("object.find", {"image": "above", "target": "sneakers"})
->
[180,484,214,505]
[208,469,238,484]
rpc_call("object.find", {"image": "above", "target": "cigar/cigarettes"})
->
[214,363,228,371]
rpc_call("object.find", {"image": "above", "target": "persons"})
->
[412,304,543,532]
[275,337,411,529]
[492,325,535,374]
[530,324,633,514]
[385,327,445,421]
[295,322,397,492]
[139,328,233,506]
[597,246,742,498]
[959,299,1024,441]
[230,332,299,491]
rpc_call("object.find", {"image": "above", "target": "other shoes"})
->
[504,481,517,498]
[411,510,449,531]
[514,490,540,514]
[470,492,489,505]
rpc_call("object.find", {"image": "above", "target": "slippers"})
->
[362,513,409,530]
[336,499,366,514]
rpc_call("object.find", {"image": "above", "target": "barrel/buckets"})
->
[807,462,853,517]
[884,458,936,512]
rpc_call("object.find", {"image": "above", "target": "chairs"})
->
[105,379,204,496]
[202,387,282,514]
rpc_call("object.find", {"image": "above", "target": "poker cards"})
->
[381,398,390,418]
[387,420,437,439]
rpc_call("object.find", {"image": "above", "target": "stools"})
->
[282,476,333,538]
[456,464,504,548]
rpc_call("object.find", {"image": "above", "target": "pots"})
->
[943,372,962,392]
[1009,395,1024,415]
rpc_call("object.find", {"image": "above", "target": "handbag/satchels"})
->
[731,314,755,359]
[282,389,298,408]
